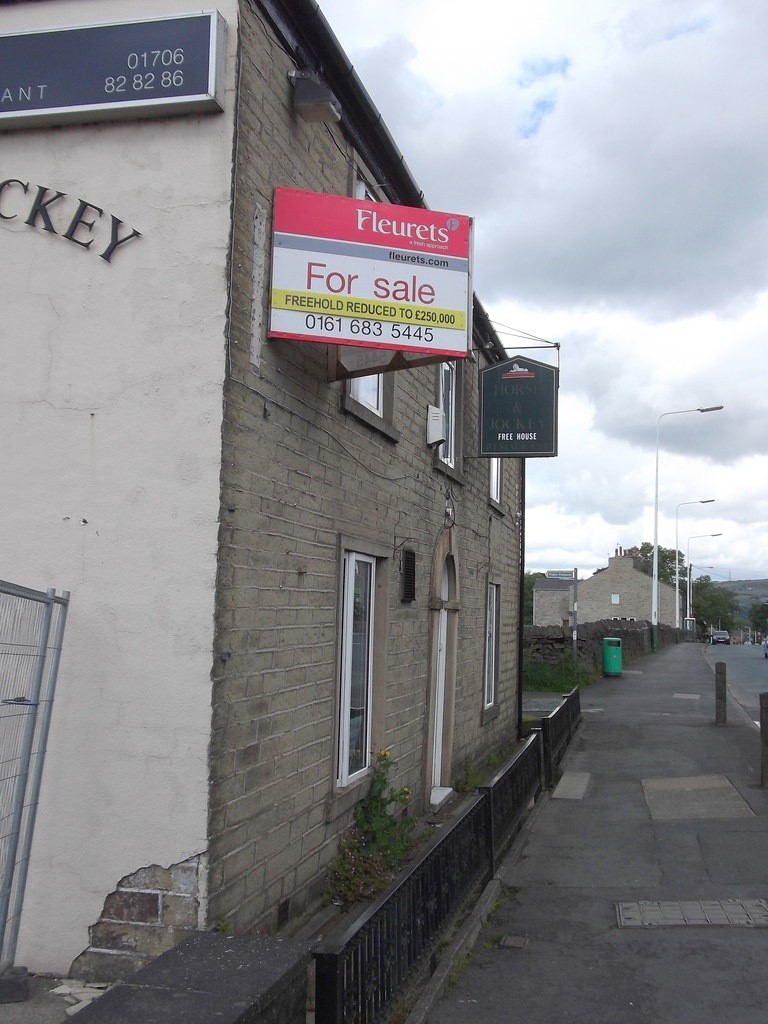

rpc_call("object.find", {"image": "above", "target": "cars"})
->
[711,630,730,646]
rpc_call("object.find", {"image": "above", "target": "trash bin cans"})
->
[603,637,623,678]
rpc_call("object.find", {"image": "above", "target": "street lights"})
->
[691,565,714,641]
[674,500,716,641]
[685,532,722,639]
[653,405,725,651]
[738,625,752,645]
